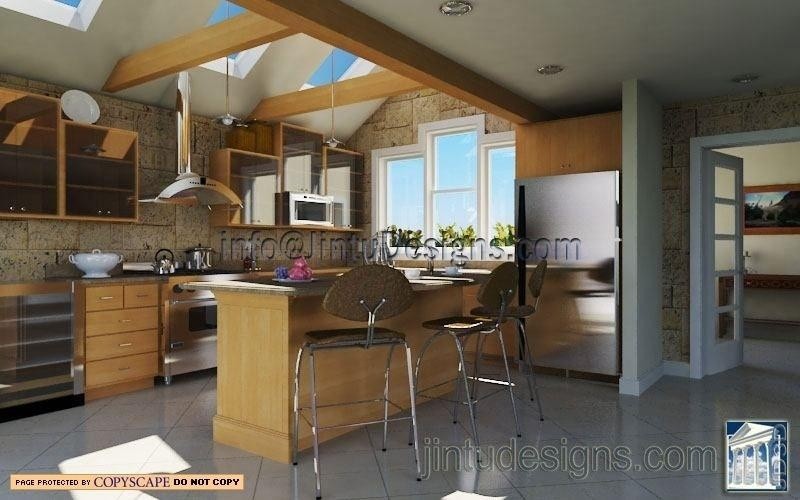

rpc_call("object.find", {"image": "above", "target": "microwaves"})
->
[284,191,335,226]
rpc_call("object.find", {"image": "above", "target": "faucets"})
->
[249,230,262,269]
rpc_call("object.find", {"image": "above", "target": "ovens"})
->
[168,301,220,375]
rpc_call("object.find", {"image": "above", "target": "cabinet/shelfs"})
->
[0,86,140,221]
[79,283,163,402]
[209,120,365,233]
[515,108,622,176]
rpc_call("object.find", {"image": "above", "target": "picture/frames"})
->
[742,183,800,235]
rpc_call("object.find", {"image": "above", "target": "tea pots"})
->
[150,248,177,275]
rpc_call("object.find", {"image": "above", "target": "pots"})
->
[184,242,217,271]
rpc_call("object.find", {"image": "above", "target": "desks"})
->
[716,274,800,338]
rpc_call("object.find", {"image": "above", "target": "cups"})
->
[444,266,459,274]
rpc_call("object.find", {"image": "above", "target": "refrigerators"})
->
[510,169,623,379]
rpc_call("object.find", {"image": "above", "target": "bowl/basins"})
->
[404,268,421,278]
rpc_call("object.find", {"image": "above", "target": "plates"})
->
[61,88,101,124]
[271,277,318,283]
[406,275,425,281]
[441,272,466,277]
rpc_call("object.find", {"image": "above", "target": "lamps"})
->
[214,1,247,127]
[320,46,344,148]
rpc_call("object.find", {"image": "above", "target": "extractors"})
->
[138,70,244,211]
